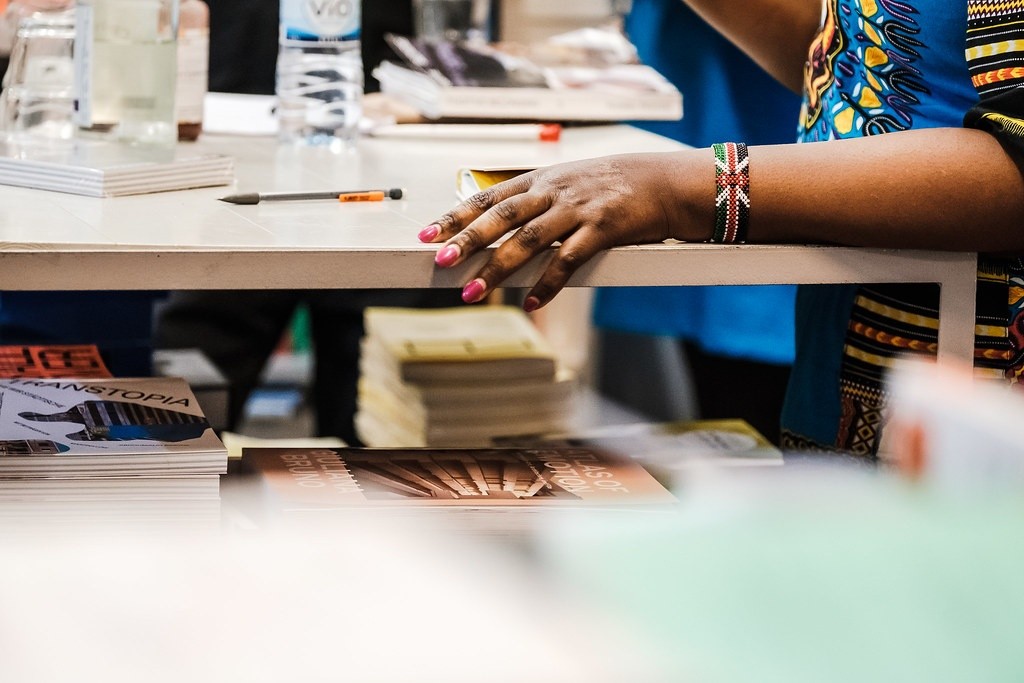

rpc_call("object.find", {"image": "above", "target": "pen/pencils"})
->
[364,120,562,141]
[220,187,403,206]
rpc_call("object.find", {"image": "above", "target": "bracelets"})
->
[712,141,750,243]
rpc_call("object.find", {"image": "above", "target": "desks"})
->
[0,91,978,380]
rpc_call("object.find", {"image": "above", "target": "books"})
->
[352,304,583,448]
[372,29,685,122]
[0,342,785,501]
[0,133,235,198]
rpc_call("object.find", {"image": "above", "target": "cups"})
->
[3,19,77,132]
[90,1,179,141]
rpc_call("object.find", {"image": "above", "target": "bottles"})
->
[274,0,363,148]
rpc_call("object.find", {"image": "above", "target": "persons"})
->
[418,0,1024,456]
